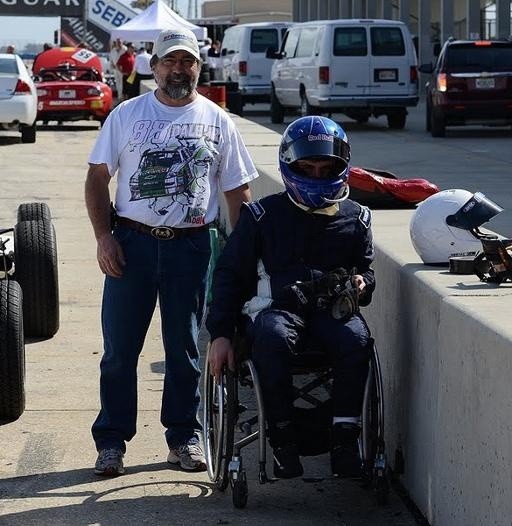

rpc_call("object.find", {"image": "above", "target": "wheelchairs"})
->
[201,223,392,508]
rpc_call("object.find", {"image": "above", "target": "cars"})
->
[0,47,116,143]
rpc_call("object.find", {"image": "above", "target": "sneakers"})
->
[94,449,125,476]
[331,436,362,478]
[167,444,208,472]
[272,447,303,479]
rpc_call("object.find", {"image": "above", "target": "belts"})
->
[123,219,209,241]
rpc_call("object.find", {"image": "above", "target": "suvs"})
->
[424,35,512,139]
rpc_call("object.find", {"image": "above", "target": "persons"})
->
[110,38,221,102]
[7,45,15,53]
[32,43,52,71]
[85,29,259,477]
[205,116,376,479]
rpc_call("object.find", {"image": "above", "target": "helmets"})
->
[409,188,504,266]
[278,115,351,210]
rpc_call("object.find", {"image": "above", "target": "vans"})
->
[208,20,419,130]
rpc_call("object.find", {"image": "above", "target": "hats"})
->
[149,29,201,68]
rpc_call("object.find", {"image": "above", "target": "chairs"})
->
[42,72,57,81]
[80,73,96,81]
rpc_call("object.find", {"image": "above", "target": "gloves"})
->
[282,268,359,321]
